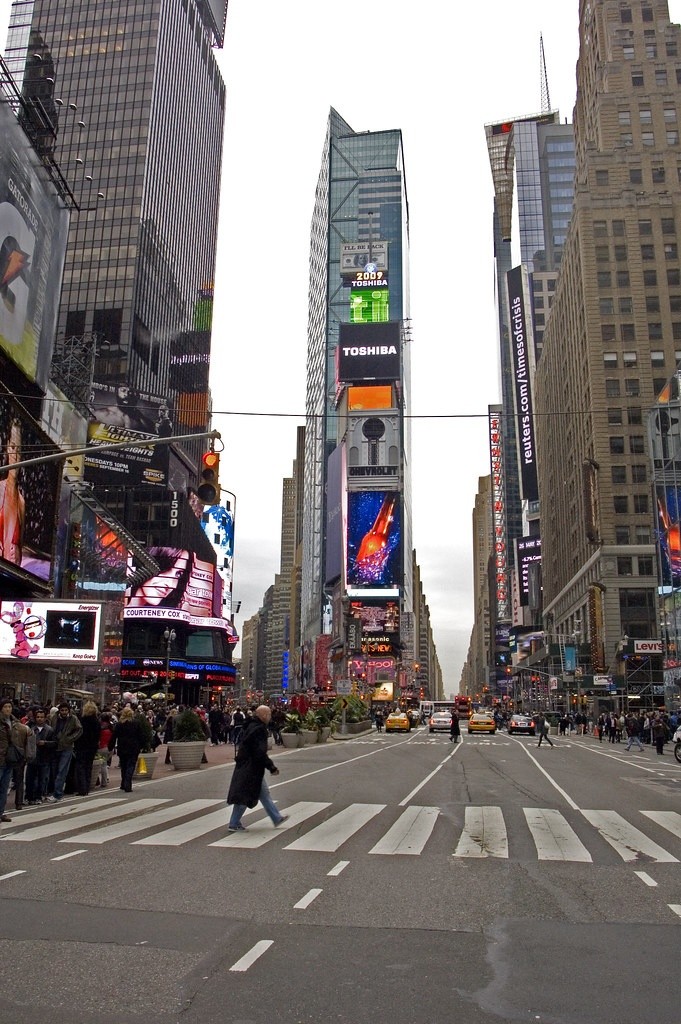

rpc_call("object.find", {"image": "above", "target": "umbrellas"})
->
[134,691,176,700]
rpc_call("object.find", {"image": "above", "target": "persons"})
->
[88,381,172,488]
[0,695,292,822]
[449,709,460,744]
[537,711,554,749]
[593,704,681,755]
[125,546,194,610]
[470,700,594,736]
[371,701,427,733]
[356,254,366,268]
[0,417,26,567]
[226,705,290,833]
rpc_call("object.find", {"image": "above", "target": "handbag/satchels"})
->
[135,757,147,776]
[544,720,550,729]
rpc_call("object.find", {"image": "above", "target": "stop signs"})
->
[256,691,264,698]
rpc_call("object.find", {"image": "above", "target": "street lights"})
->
[164,627,177,713]
[217,687,222,711]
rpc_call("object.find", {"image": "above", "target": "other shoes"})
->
[0,815,11,823]
[449,737,459,743]
[16,794,64,811]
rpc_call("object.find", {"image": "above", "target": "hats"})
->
[58,703,70,709]
[0,699,12,709]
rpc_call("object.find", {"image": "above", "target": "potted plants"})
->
[167,709,207,771]
[89,754,103,789]
[132,713,159,781]
[279,707,336,748]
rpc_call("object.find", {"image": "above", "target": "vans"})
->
[533,711,562,721]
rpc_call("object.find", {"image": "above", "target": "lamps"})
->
[64,175,93,181]
[54,159,83,164]
[65,459,73,464]
[2,53,43,62]
[52,121,86,128]
[34,103,77,111]
[26,98,63,106]
[232,600,241,613]
[63,466,79,472]
[13,77,55,85]
[72,192,104,198]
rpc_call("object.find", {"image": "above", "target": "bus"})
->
[270,693,337,713]
[419,701,486,717]
[455,695,473,720]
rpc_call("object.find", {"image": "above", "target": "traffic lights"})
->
[360,695,366,700]
[197,453,219,507]
[507,668,511,673]
[353,682,359,690]
[415,664,419,672]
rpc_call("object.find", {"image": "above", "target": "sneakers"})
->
[275,816,289,827]
[228,826,249,833]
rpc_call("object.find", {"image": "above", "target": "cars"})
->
[410,710,419,721]
[672,725,681,763]
[468,713,496,734]
[429,712,452,733]
[508,714,535,736]
[386,709,410,733]
